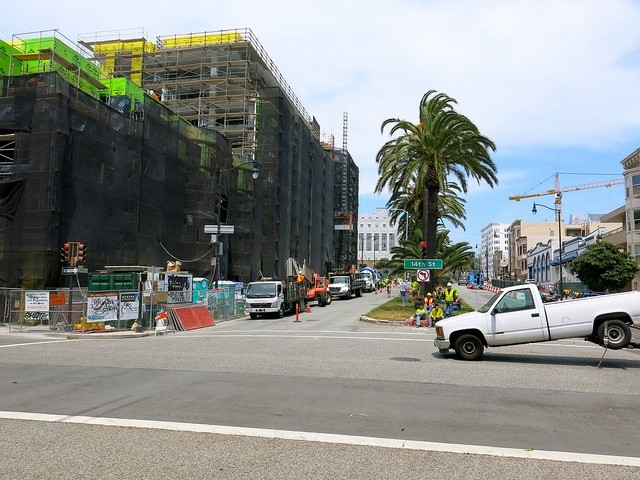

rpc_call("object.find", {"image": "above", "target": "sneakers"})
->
[416,325,419,327]
[413,314,417,318]
[428,326,431,327]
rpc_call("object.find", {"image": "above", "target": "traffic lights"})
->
[420,240,428,259]
[60,241,88,267]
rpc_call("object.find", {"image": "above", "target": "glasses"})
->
[447,286,451,287]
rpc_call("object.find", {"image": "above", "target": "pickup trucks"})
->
[434,283,607,361]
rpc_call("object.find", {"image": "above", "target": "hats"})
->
[433,304,439,308]
[426,292,432,296]
[447,282,451,286]
[299,271,302,274]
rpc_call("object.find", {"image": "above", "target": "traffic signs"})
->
[62,266,79,276]
[416,268,431,283]
[403,258,444,270]
[499,255,509,278]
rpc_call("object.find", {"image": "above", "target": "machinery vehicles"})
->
[306,273,332,308]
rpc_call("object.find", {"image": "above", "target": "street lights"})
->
[215,161,263,289]
[531,201,563,301]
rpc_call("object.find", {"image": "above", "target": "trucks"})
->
[361,272,381,292]
[245,277,307,319]
[326,272,365,300]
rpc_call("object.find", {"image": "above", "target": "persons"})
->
[386,281,392,298]
[560,288,571,301]
[446,293,461,315]
[428,304,444,328]
[573,291,580,298]
[423,292,436,314]
[444,281,454,317]
[411,279,420,301]
[413,303,428,328]
[400,283,408,304]
